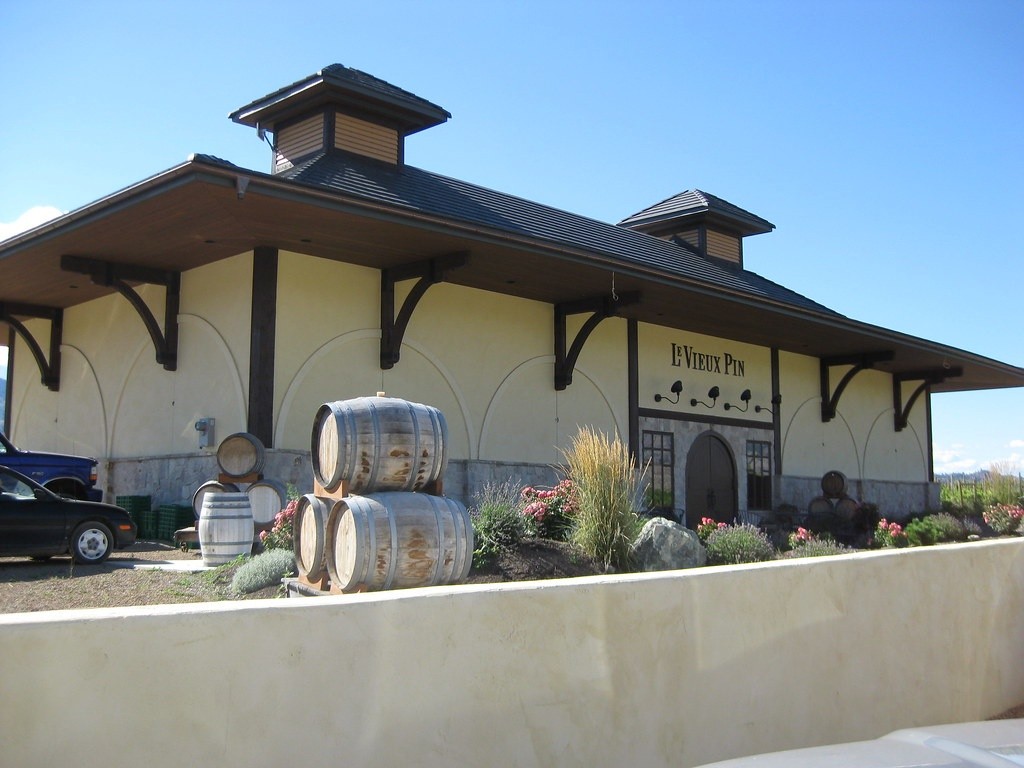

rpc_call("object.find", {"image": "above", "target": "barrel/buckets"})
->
[245,479,290,533]
[198,492,254,567]
[293,493,338,584]
[324,491,474,591]
[310,396,450,495]
[217,433,267,479]
[808,470,859,521]
[191,480,241,520]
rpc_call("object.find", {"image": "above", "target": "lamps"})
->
[691,387,720,408]
[655,380,683,404]
[724,389,751,412]
[755,395,782,415]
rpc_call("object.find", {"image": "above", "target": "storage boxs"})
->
[115,495,200,549]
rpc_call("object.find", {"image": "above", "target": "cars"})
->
[0,466,138,565]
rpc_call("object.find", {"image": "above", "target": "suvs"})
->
[0,431,102,501]
